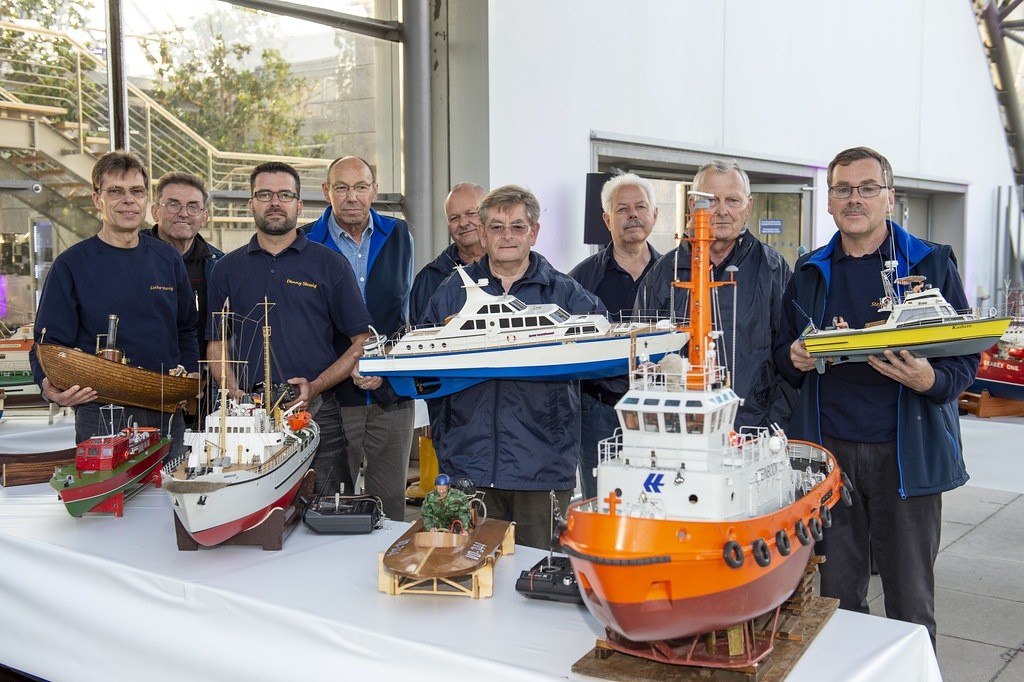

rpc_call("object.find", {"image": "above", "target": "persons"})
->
[350,186,627,550]
[407,182,488,483]
[206,162,371,501]
[28,150,201,466]
[560,175,664,501]
[419,473,472,537]
[136,171,226,438]
[777,147,982,656]
[296,156,416,527]
[638,157,806,443]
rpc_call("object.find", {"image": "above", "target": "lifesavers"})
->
[723,541,744,568]
[125,451,128,457]
[809,518,823,541]
[776,529,791,555]
[841,472,854,492]
[820,505,832,528]
[753,538,771,567]
[249,393,260,403]
[795,520,810,545]
[990,307,997,316]
[507,334,516,343]
[882,296,892,307]
[840,485,852,507]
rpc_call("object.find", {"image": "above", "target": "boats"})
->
[356,265,691,401]
[160,294,321,546]
[49,404,173,518]
[33,315,206,415]
[966,275,1024,401]
[557,189,845,667]
[798,166,1014,375]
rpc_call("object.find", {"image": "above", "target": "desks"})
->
[0,407,76,488]
[958,412,1024,494]
[0,482,946,682]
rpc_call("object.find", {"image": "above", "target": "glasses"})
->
[329,180,374,194]
[251,190,299,202]
[486,222,528,236]
[100,186,148,200]
[158,201,204,216]
[829,183,890,199]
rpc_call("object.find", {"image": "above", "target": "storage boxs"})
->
[960,390,1024,419]
